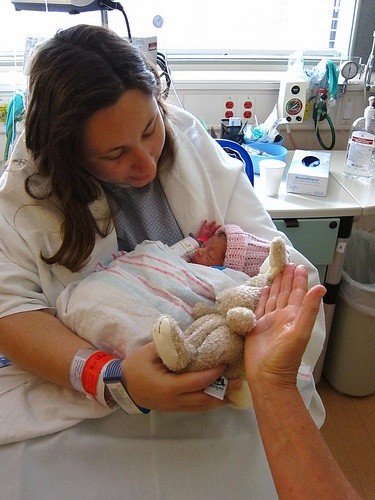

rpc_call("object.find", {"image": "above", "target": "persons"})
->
[170,219,271,277]
[245,263,362,500]
[0,24,294,408]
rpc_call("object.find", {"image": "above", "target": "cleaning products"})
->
[342,95,375,184]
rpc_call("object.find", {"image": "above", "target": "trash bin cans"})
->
[324,230,374,396]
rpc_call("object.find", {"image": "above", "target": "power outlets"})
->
[239,95,256,124]
[222,95,239,118]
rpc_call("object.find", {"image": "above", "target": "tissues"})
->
[286,150,332,197]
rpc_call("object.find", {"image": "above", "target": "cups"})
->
[259,159,286,196]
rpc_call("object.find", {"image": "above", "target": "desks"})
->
[252,149,375,384]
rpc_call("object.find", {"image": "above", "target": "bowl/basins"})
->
[242,143,288,174]
[243,133,284,145]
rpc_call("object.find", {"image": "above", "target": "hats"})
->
[215,224,270,278]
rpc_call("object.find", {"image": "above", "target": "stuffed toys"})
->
[153,237,289,409]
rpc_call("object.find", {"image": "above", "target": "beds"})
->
[0,101,327,500]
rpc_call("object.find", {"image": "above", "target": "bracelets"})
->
[103,360,151,415]
[81,350,122,410]
[68,345,97,393]
